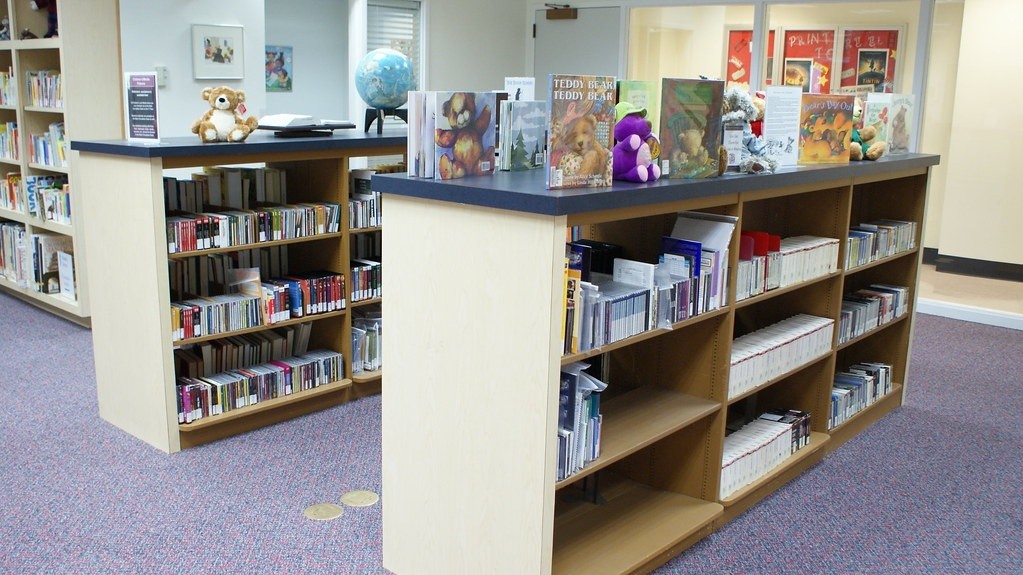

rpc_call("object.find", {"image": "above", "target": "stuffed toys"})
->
[850,97,886,161]
[723,86,780,173]
[612,102,661,182]
[191,87,258,143]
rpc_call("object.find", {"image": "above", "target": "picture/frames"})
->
[191,23,245,80]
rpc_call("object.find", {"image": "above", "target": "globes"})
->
[354,48,415,135]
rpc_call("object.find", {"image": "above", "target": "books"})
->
[408,74,915,190]
[556,218,918,500]
[162,162,407,424]
[0,66,78,302]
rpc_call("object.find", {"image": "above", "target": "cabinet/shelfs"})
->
[0,0,126,329]
[69,129,408,454]
[371,152,941,575]
